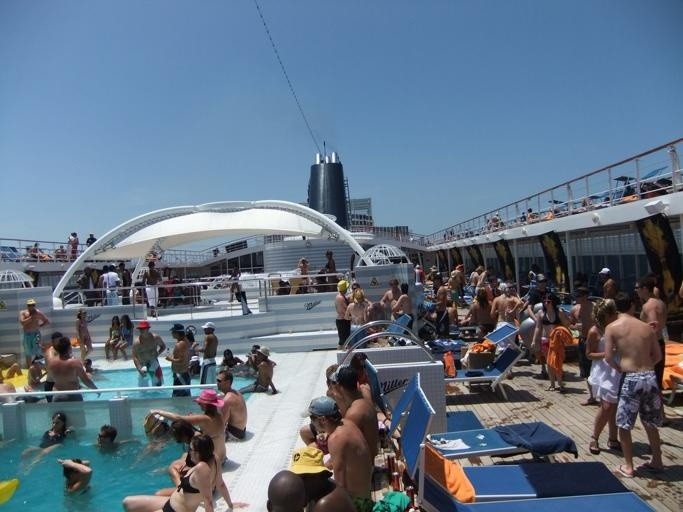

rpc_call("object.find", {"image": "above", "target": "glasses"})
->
[216,379,224,383]
[310,415,317,420]
[633,286,641,291]
[28,305,35,307]
[542,300,551,304]
[538,280,547,283]
[188,447,197,451]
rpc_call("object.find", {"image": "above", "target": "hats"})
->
[256,346,271,356]
[498,282,508,292]
[148,261,155,266]
[308,396,338,416]
[143,414,160,437]
[169,323,186,332]
[193,389,225,408]
[536,273,548,282]
[598,267,610,274]
[289,447,333,477]
[337,280,350,292]
[27,298,37,305]
[201,322,216,328]
[329,363,357,385]
[136,321,151,329]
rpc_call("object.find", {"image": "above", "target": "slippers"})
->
[615,465,633,478]
[640,462,664,472]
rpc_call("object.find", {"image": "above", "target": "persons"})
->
[443,182,642,241]
[0,299,276,512]
[267,351,400,511]
[298,250,338,292]
[335,263,682,479]
[77,262,162,318]
[24,233,97,264]
[229,265,241,302]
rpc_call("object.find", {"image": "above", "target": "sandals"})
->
[589,436,600,454]
[606,439,621,450]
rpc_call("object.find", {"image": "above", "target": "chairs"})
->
[289,278,303,294]
[339,358,438,510]
[385,313,525,402]
[424,420,654,511]
[0,246,84,264]
[266,273,281,295]
